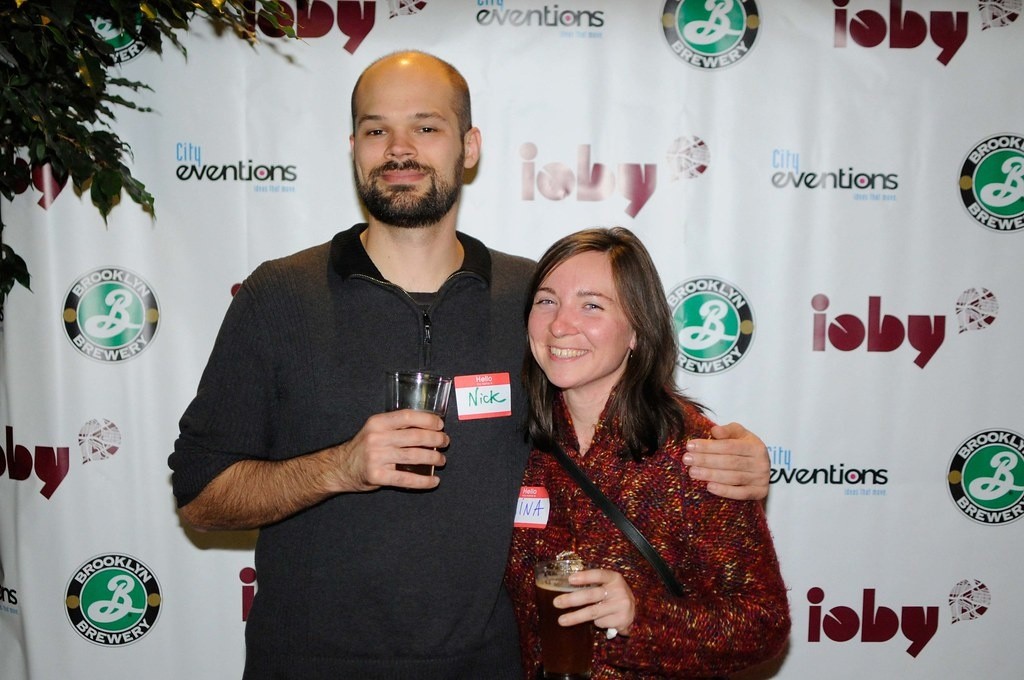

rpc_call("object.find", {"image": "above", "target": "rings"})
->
[602,586,608,601]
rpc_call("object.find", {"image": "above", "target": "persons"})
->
[503,226,790,680]
[166,49,770,680]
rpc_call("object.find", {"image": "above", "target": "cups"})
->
[534,558,597,680]
[385,370,453,477]
